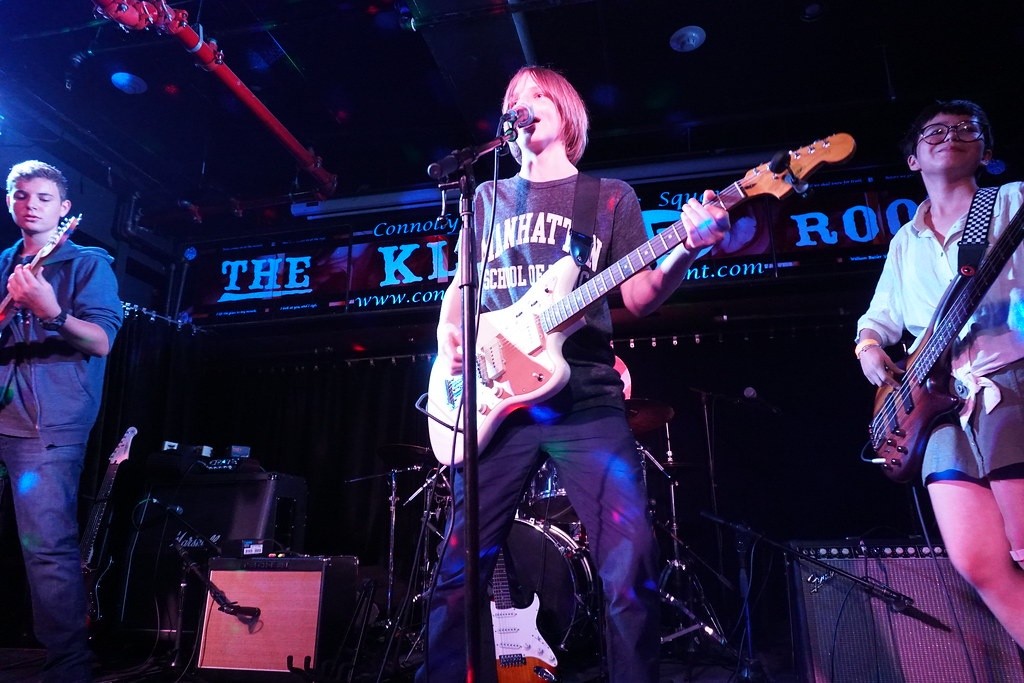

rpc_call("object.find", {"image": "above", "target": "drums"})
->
[473,508,595,660]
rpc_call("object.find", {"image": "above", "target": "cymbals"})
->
[623,395,679,432]
[647,459,709,477]
[399,444,438,462]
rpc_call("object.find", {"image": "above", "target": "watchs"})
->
[34,308,68,332]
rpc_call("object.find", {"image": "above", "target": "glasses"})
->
[911,120,987,155]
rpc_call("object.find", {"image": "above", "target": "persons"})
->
[411,65,732,682]
[0,159,124,683]
[854,99,1024,650]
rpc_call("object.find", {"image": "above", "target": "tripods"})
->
[349,464,429,654]
[657,417,750,665]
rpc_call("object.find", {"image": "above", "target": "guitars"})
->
[0,213,82,328]
[488,548,559,683]
[527,452,579,526]
[425,130,856,469]
[77,427,141,629]
[868,202,1023,479]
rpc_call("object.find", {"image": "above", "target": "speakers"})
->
[124,473,360,682]
[787,538,1024,683]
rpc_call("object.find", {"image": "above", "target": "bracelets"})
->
[856,338,878,357]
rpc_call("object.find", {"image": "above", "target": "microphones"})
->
[220,605,262,618]
[889,596,915,613]
[501,102,535,126]
